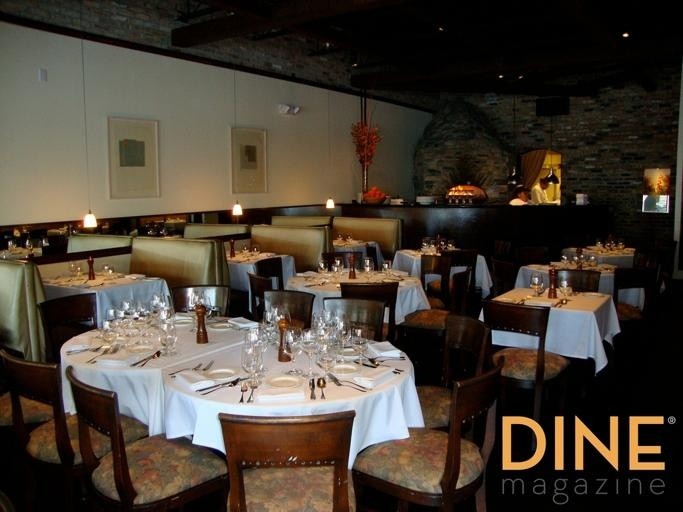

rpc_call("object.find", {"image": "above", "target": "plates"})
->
[204,365,239,380]
[175,318,192,328]
[208,319,236,331]
[415,196,434,206]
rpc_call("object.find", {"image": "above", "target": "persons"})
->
[509,185,535,207]
[531,177,550,205]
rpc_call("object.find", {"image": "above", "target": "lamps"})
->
[544,115,561,185]
[232,8,243,216]
[325,39,335,209]
[80,1,98,229]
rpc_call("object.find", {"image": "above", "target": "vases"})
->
[362,162,368,195]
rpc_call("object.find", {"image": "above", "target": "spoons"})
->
[200,377,239,395]
[317,377,327,399]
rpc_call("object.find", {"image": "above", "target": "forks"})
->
[170,359,215,378]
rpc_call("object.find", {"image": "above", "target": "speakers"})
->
[536,96,569,115]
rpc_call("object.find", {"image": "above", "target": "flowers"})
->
[349,110,384,165]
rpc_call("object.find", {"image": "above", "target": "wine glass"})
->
[186,289,213,333]
[239,301,406,386]
[241,242,260,260]
[529,240,626,304]
[147,227,168,237]
[68,260,83,280]
[421,230,455,258]
[317,256,393,284]
[69,292,179,357]
[102,262,117,280]
[0,227,50,260]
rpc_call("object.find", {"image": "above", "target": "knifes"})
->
[195,376,247,392]
[308,378,316,399]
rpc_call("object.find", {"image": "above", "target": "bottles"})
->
[229,238,236,258]
[86,255,97,281]
[194,304,209,344]
